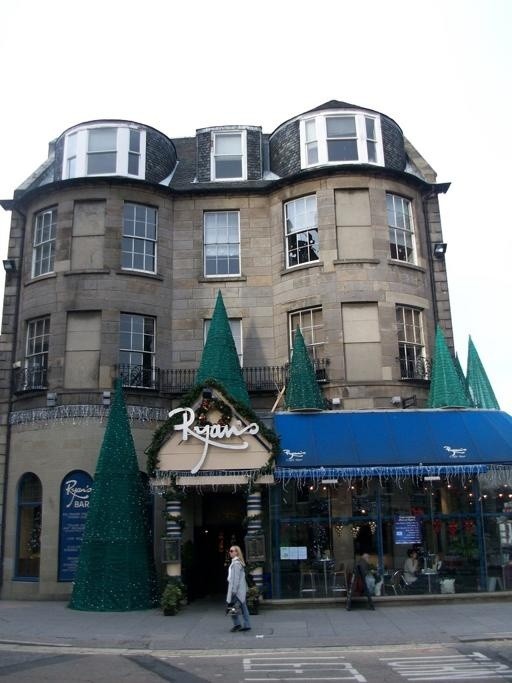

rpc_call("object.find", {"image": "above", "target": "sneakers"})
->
[230,625,241,631]
[239,628,251,631]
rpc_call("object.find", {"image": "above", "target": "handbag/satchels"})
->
[245,573,256,588]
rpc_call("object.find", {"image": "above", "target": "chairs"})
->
[297,554,439,595]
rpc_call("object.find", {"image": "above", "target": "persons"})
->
[225,545,251,632]
[403,549,427,589]
[359,552,375,578]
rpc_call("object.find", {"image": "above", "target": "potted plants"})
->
[245,586,261,613]
[160,576,188,615]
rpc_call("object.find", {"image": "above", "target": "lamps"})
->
[245,536,266,563]
[161,536,183,564]
